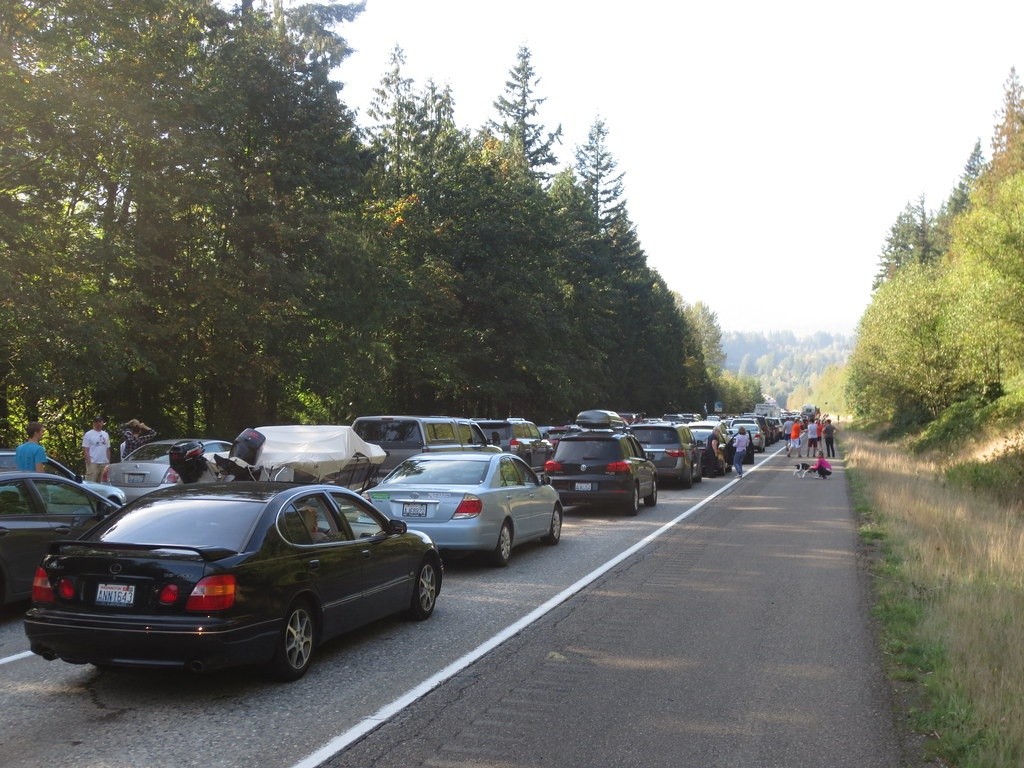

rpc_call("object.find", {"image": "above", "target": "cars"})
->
[23,482,445,685]
[0,448,128,514]
[537,403,820,478]
[0,468,121,623]
[354,451,563,568]
[101,439,233,503]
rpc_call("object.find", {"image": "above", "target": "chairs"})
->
[0,490,20,514]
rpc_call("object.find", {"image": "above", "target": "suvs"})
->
[543,410,658,517]
[470,417,555,475]
[629,420,704,490]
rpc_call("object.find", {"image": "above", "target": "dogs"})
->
[793,463,817,479]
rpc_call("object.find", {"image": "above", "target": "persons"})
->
[15,422,48,472]
[809,451,832,480]
[120,419,157,460]
[804,418,824,457]
[82,417,112,485]
[822,419,840,458]
[732,427,749,478]
[782,417,804,458]
[706,426,720,478]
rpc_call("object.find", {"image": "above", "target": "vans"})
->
[349,416,503,488]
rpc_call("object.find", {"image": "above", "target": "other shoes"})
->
[786,448,789,451]
[825,455,830,458]
[734,473,742,478]
[787,453,790,457]
[814,474,829,480]
[798,455,801,457]
